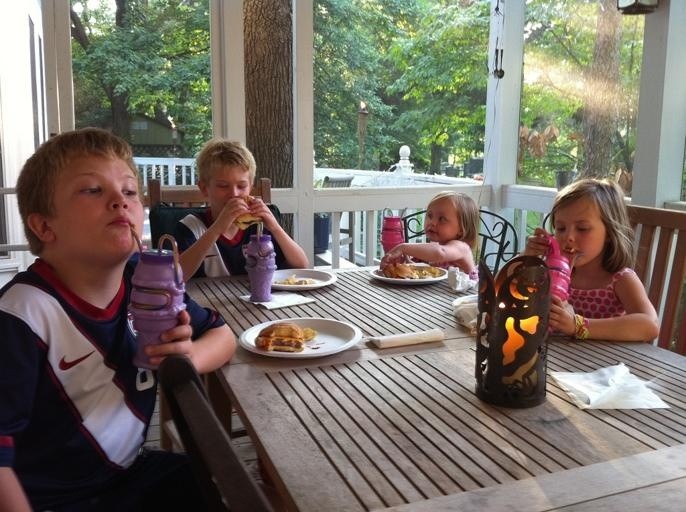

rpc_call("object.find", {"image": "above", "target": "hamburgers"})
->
[233,195,261,230]
[255,322,305,353]
[379,256,411,278]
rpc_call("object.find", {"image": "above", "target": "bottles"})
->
[542,252,571,334]
[245,234,277,303]
[130,247,186,371]
[380,216,406,256]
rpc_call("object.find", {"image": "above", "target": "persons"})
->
[0,126,237,512]
[381,192,480,281]
[160,138,309,279]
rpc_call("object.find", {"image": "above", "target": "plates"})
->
[370,264,447,286]
[238,317,360,360]
[269,268,337,291]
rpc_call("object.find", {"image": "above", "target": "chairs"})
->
[321,176,355,234]
[627,204,685,355]
[159,356,274,511]
[149,177,282,250]
[401,210,518,278]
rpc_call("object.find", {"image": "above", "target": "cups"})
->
[554,170,571,191]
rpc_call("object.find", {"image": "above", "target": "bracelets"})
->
[524,177,661,341]
[574,314,590,342]
